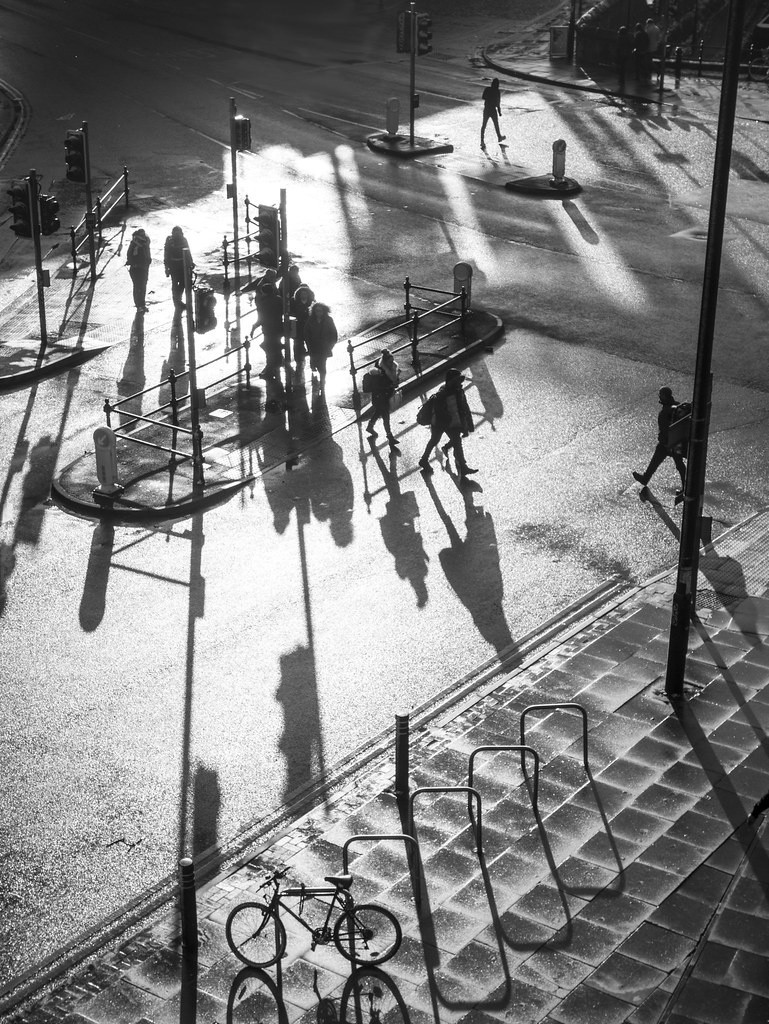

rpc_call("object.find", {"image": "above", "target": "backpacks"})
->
[361,368,380,392]
[416,394,437,425]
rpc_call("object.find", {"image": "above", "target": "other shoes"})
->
[675,488,683,494]
[419,458,433,472]
[480,143,486,147]
[498,135,506,143]
[366,427,378,436]
[442,446,450,458]
[458,465,478,475]
[633,472,649,486]
[388,438,399,444]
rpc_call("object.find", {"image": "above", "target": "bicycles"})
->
[748,58,769,82]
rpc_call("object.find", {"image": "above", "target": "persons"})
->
[481,77,506,148]
[614,17,663,90]
[166,225,194,312]
[366,349,401,446]
[632,386,687,496]
[125,226,151,315]
[254,263,339,379]
[418,369,479,478]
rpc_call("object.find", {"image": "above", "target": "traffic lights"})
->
[40,195,60,237]
[64,129,89,186]
[253,204,279,270]
[193,288,217,335]
[5,179,33,238]
[417,13,433,57]
